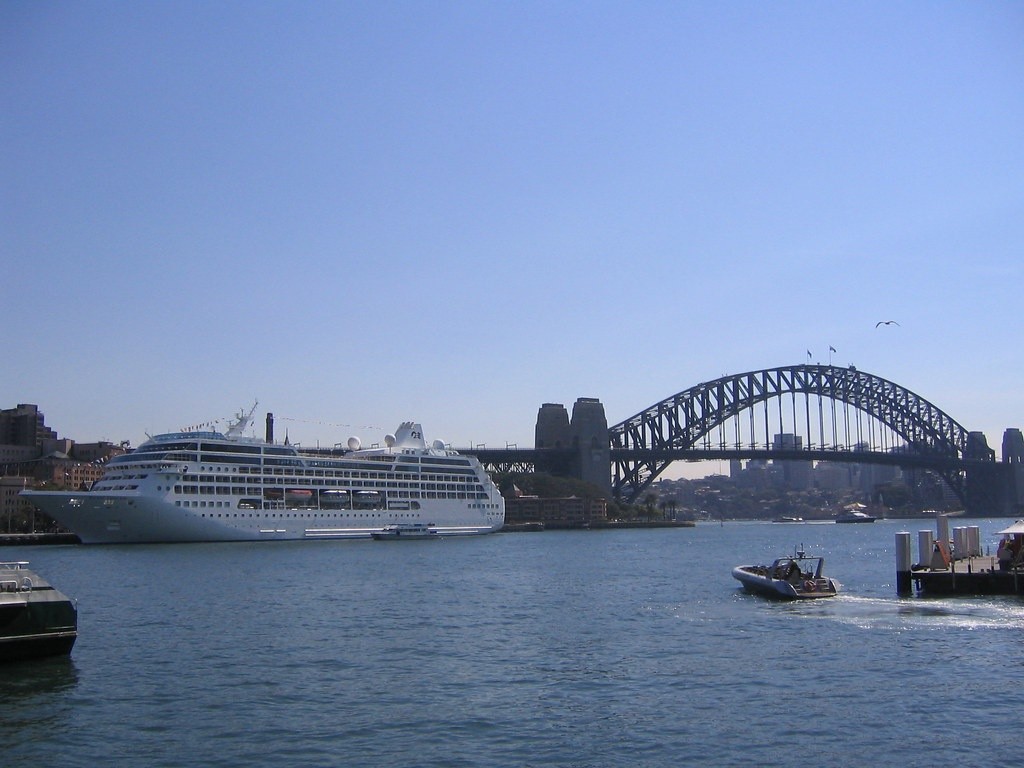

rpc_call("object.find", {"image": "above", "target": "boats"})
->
[0,559,78,662]
[320,489,349,504]
[370,523,444,541]
[732,542,844,601]
[352,490,382,504]
[772,515,805,523]
[835,505,878,522]
[285,489,312,502]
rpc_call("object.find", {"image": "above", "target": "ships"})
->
[19,401,505,545]
